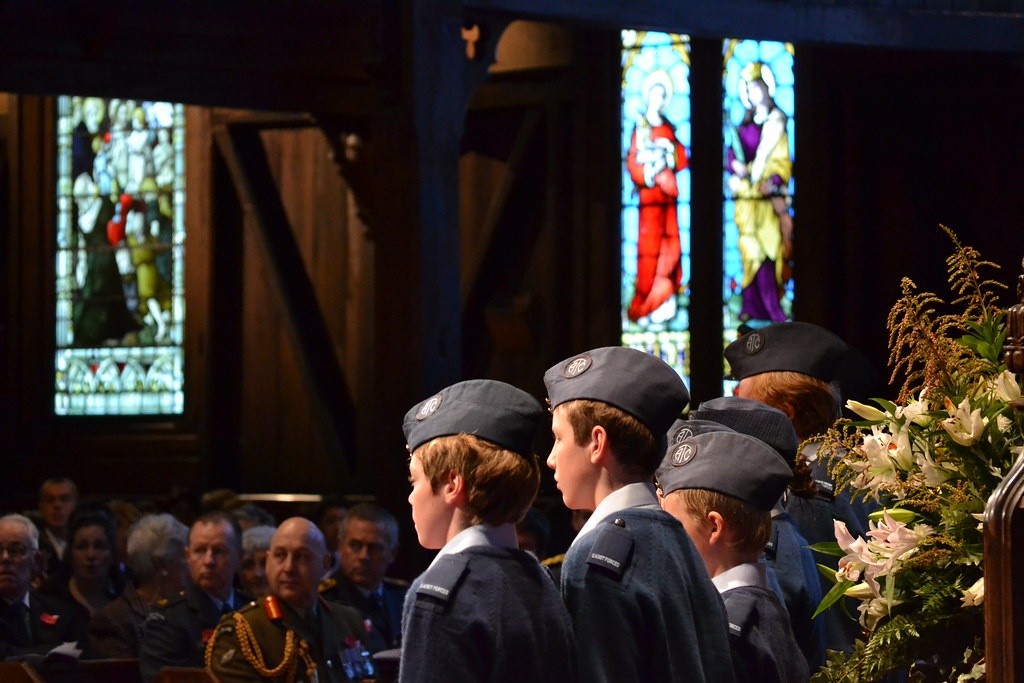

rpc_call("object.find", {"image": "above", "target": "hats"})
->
[654,396,797,511]
[402,379,545,457]
[723,321,855,385]
[542,347,690,441]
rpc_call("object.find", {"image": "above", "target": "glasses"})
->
[0,541,37,559]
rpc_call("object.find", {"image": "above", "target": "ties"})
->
[369,590,391,642]
[303,607,322,656]
[10,602,34,646]
[220,602,233,619]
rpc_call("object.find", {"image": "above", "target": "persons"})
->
[397,379,580,683]
[0,478,403,683]
[655,324,864,683]
[544,346,736,683]
